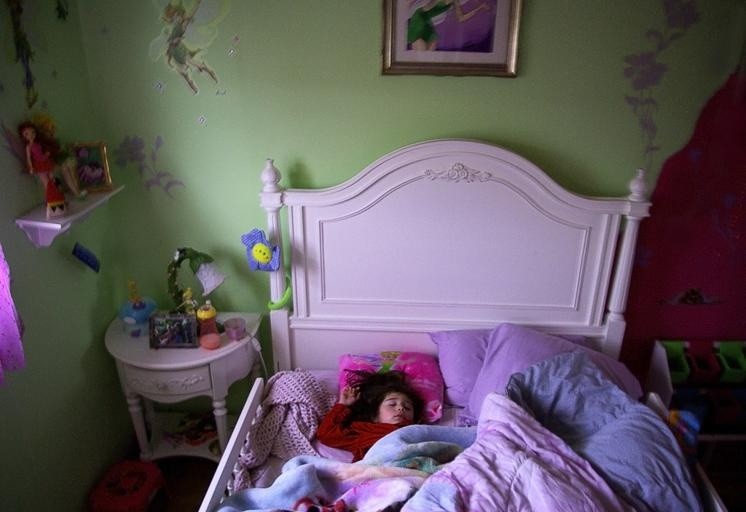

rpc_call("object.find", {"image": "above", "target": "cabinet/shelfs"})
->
[645,333,746,448]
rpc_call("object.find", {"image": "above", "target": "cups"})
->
[224,318,246,340]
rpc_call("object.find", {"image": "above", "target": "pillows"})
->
[431,324,598,407]
[339,348,446,419]
[467,321,644,424]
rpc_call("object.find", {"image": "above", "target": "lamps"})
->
[166,246,229,310]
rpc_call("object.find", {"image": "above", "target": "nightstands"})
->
[106,311,266,465]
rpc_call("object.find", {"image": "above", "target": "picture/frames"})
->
[380,0,522,82]
[70,139,114,194]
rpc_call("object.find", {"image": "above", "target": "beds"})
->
[197,133,722,512]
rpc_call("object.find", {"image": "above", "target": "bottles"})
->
[197,304,221,349]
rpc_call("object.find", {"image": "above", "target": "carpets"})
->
[76,442,216,511]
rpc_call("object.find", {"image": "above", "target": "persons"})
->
[39,120,90,200]
[313,366,423,464]
[20,123,54,198]
